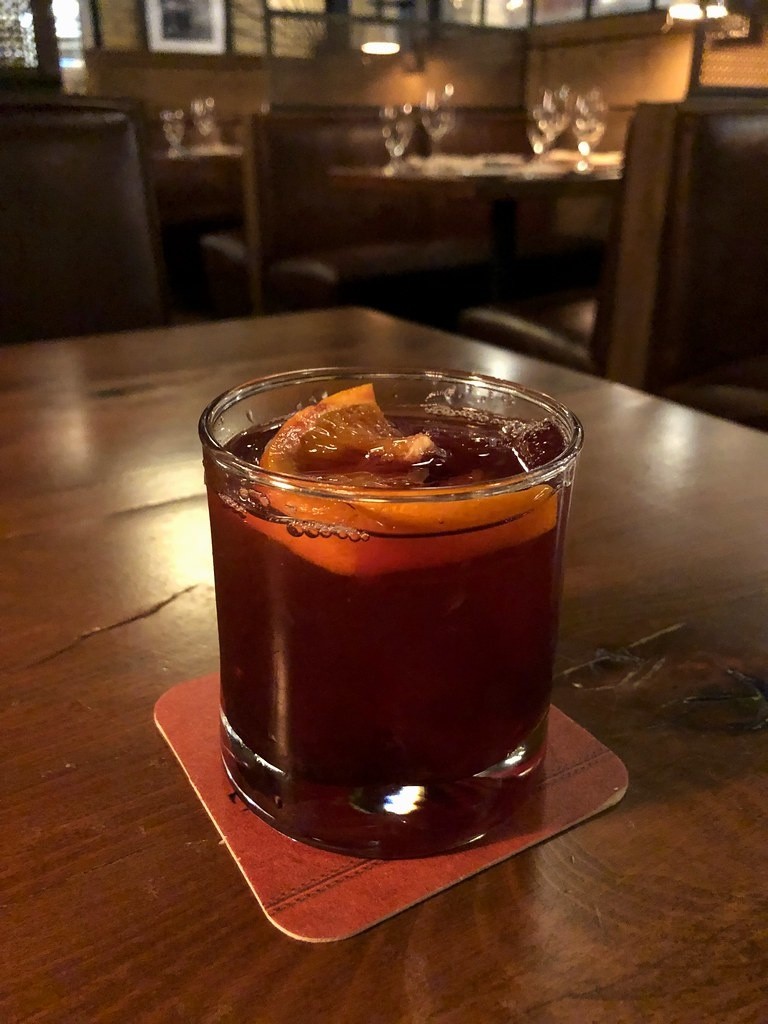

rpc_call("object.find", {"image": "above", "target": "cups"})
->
[198,365,582,855]
[525,111,555,163]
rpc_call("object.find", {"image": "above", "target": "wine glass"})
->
[192,98,215,148]
[163,111,184,156]
[572,100,608,172]
[379,104,415,170]
[535,85,573,160]
[420,86,455,157]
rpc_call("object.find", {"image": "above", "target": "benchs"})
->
[0,95,768,434]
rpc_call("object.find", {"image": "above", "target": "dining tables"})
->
[325,149,620,301]
[146,133,240,224]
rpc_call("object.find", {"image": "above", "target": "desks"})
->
[0,306,768,1024]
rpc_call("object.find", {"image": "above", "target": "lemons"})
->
[266,385,557,564]
